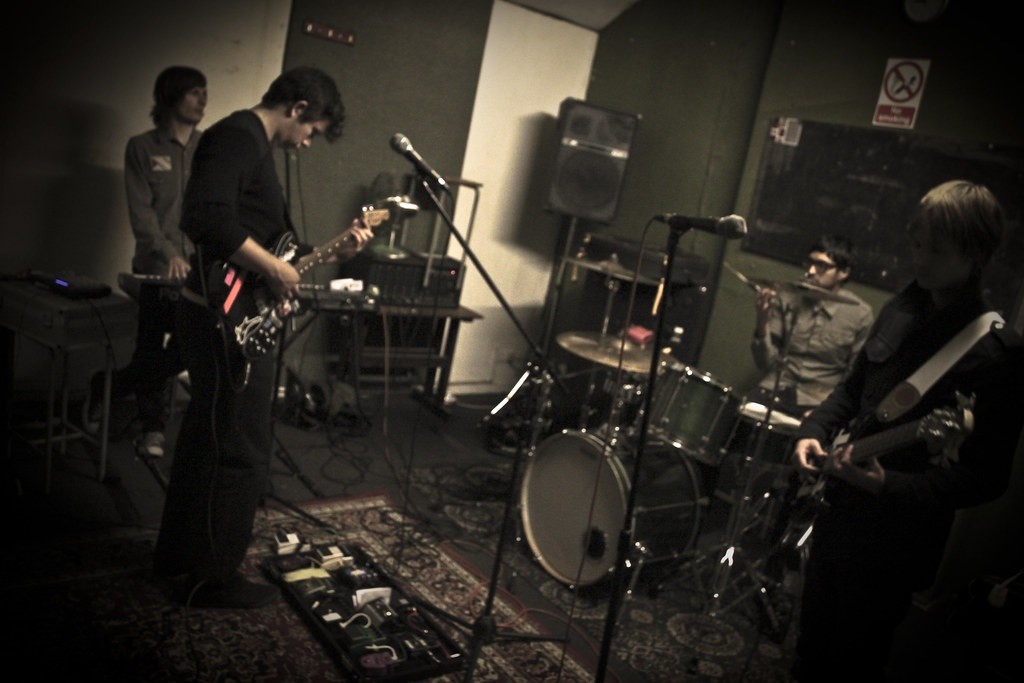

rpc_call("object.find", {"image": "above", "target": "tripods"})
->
[413,174,571,683]
[644,295,805,676]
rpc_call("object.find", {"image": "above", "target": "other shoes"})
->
[180,575,280,609]
[131,431,165,457]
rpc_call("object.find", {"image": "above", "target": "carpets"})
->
[407,459,805,683]
[0,488,619,683]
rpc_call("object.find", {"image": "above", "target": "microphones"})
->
[655,210,747,239]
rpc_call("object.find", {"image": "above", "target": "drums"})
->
[711,401,802,514]
[644,359,744,467]
[520,419,706,591]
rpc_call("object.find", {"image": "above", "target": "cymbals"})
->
[555,329,677,378]
[563,255,660,286]
[747,274,860,307]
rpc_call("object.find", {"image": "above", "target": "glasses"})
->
[801,256,839,274]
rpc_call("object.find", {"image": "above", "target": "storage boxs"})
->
[0,279,141,353]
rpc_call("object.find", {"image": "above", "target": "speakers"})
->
[539,98,642,225]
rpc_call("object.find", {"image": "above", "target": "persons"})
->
[153,66,375,611]
[82,66,206,458]
[786,179,1024,683]
[695,231,874,515]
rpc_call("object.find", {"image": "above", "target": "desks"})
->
[303,289,486,428]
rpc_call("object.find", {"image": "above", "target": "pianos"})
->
[116,267,385,499]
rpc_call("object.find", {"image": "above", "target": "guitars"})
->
[766,397,977,524]
[211,196,420,363]
